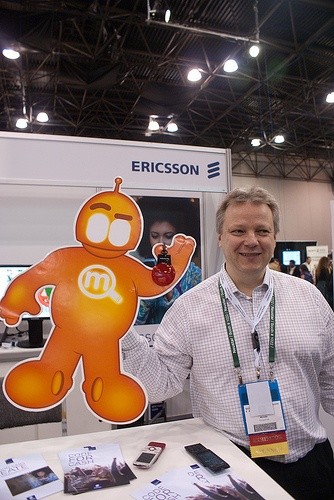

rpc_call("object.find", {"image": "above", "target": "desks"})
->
[0,418,296,500]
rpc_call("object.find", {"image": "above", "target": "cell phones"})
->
[185,443,230,472]
[133,441,165,470]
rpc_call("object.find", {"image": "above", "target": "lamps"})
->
[155,7,172,23]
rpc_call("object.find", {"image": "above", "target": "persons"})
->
[287,260,301,278]
[303,256,315,282]
[36,471,58,485]
[121,186,334,500]
[299,264,313,283]
[315,254,333,310]
[134,209,201,325]
[269,258,288,274]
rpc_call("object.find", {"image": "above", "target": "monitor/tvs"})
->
[0,265,52,348]
[282,250,301,265]
